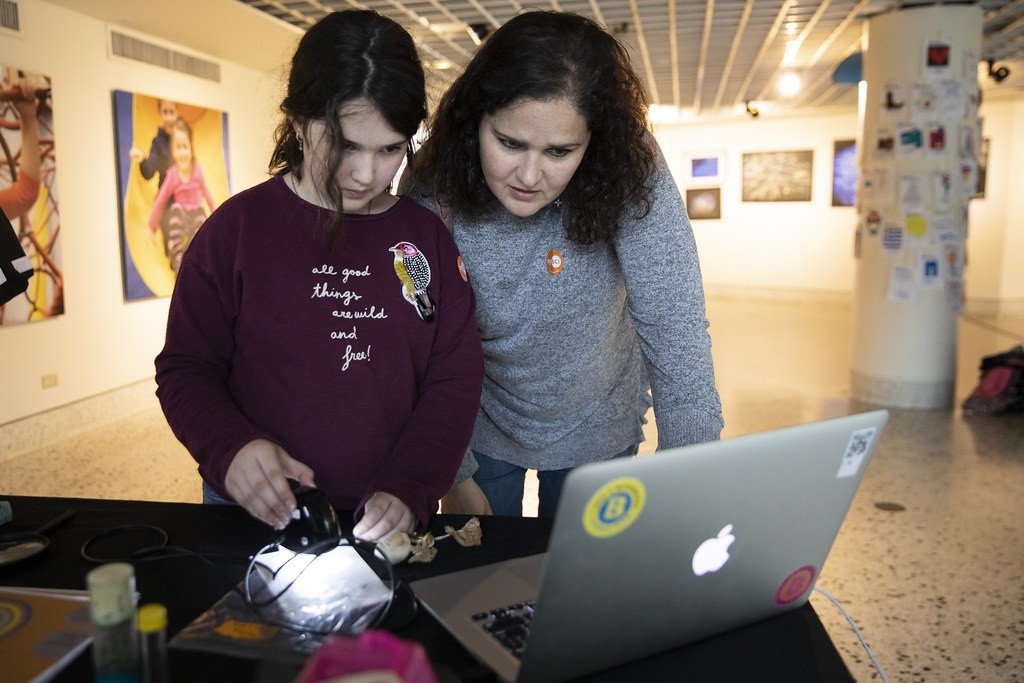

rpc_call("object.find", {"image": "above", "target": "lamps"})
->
[987,59,1008,82]
[745,100,758,117]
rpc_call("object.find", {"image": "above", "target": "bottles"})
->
[83,562,141,683]
[133,605,173,683]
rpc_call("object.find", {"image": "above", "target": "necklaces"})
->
[291,173,372,214]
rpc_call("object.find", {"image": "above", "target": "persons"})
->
[129,100,178,254]
[396,11,723,517]
[0,77,41,220]
[155,9,484,557]
[147,118,216,273]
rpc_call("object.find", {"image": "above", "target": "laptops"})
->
[407,408,890,683]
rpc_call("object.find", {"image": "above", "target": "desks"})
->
[0,496,859,683]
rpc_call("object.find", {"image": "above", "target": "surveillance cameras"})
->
[994,67,1009,82]
